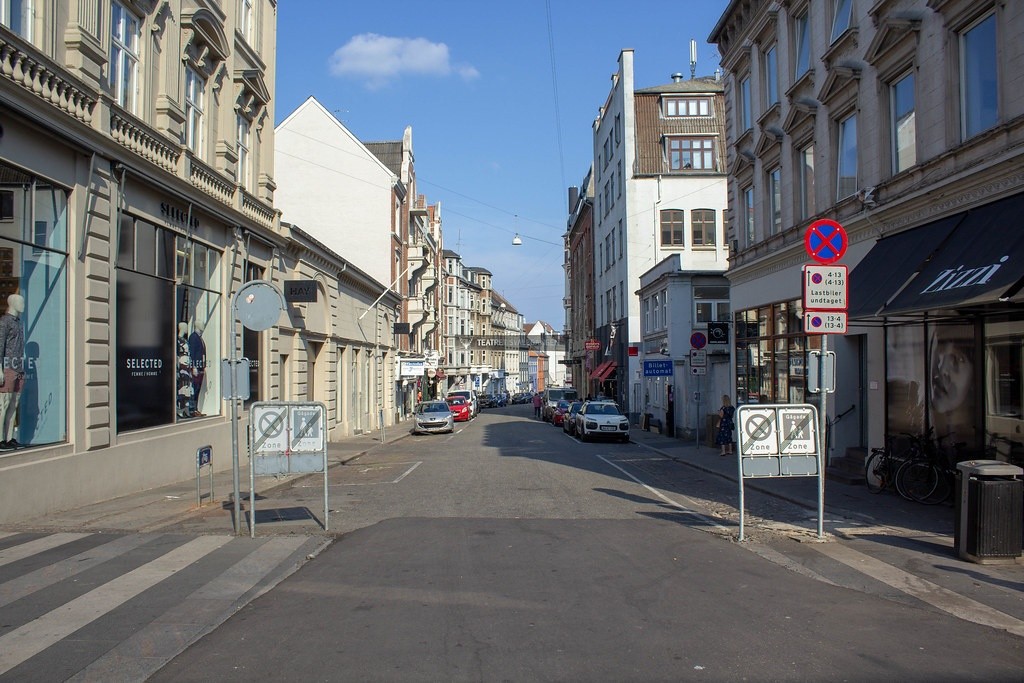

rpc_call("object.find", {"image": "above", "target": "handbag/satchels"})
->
[716,419,721,429]
[731,423,735,430]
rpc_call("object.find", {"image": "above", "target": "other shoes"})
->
[177,411,192,419]
[190,412,206,417]
[720,453,726,456]
[726,451,732,455]
[0,438,25,451]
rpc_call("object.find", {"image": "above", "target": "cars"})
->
[448,389,507,418]
[411,400,456,435]
[552,399,571,428]
[562,402,582,437]
[446,396,473,424]
[512,393,528,405]
[526,392,534,404]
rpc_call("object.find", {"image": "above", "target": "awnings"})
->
[848,186,1024,320]
[588,361,618,382]
[428,370,447,380]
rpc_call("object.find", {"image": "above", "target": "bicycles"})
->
[866,425,973,505]
[981,430,1024,467]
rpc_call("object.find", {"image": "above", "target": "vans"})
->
[539,388,578,423]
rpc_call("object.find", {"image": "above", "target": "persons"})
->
[587,393,592,400]
[759,395,768,405]
[717,395,735,456]
[458,384,465,390]
[177,322,194,418]
[507,391,510,401]
[597,392,603,398]
[928,343,976,448]
[417,392,422,403]
[0,294,27,452]
[189,320,207,417]
[533,393,542,417]
[740,386,750,403]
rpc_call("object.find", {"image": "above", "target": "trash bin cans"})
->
[953,459,1024,565]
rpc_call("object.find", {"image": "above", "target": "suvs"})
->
[577,399,632,443]
